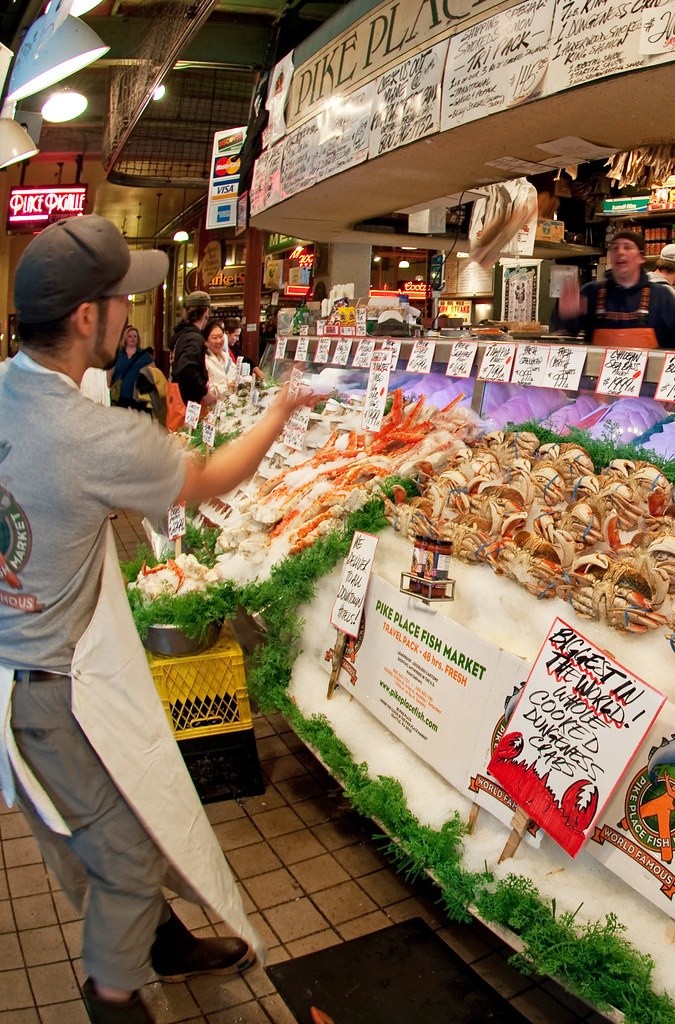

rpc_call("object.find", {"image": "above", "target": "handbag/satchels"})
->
[110,379,123,404]
[165,384,186,434]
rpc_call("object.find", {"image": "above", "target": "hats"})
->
[186,291,213,307]
[14,215,169,322]
[660,244,675,261]
[613,230,646,258]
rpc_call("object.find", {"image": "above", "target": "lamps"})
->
[173,188,190,242]
[0,0,165,170]
[399,250,410,268]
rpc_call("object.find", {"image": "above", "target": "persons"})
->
[549,232,675,352]
[165,291,267,434]
[110,328,154,409]
[0,216,337,1024]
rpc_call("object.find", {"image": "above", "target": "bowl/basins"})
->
[143,614,225,657]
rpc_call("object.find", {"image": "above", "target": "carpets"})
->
[264,916,534,1024]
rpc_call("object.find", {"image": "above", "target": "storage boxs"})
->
[138,617,267,805]
[535,218,565,244]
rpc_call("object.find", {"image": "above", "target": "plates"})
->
[377,310,403,324]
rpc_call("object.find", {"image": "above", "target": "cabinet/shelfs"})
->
[609,211,675,265]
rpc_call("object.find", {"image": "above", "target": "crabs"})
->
[139,380,675,641]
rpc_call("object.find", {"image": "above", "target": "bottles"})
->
[293,295,309,335]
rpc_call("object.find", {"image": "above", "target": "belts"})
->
[13,670,52,681]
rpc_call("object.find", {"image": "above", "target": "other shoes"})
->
[109,513,118,521]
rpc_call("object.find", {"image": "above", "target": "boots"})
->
[83,974,156,1024]
[149,905,255,982]
